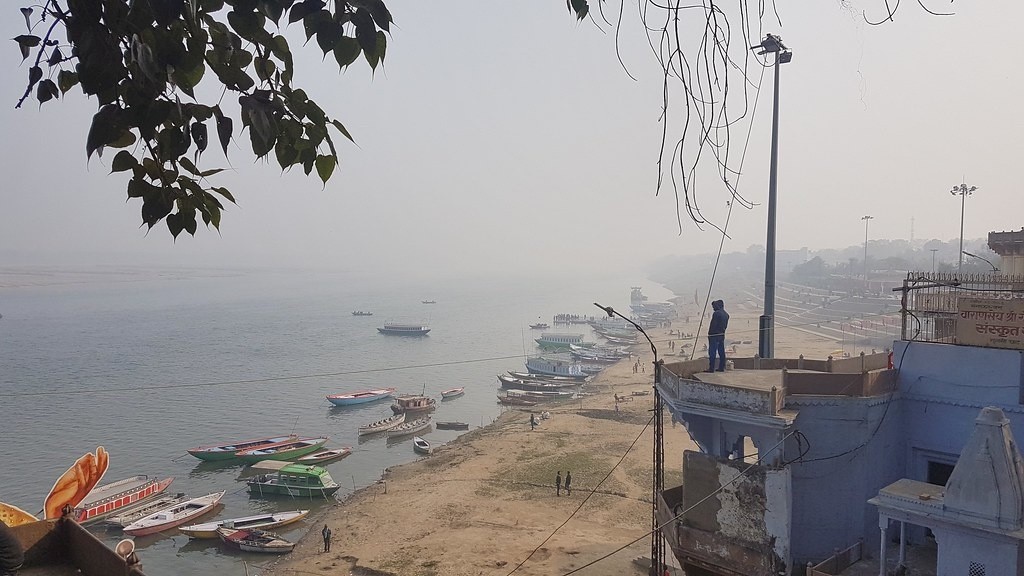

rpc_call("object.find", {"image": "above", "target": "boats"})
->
[104,491,226,536]
[376,323,432,336]
[357,411,405,435]
[185,433,300,461]
[235,435,331,463]
[412,435,430,454]
[216,524,296,554]
[392,393,436,412]
[529,324,549,328]
[325,387,397,406]
[497,332,631,406]
[298,446,353,464]
[441,386,464,398]
[436,421,468,429]
[421,300,436,303]
[352,310,372,315]
[590,286,670,345]
[179,508,311,539]
[386,415,430,435]
[248,461,342,499]
[74,474,175,529]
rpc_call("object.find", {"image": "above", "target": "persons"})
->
[556,471,561,496]
[704,300,729,372]
[614,403,619,411]
[566,470,571,496]
[530,414,534,430]
[321,523,331,553]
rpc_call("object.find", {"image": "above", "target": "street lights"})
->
[932,250,938,271]
[862,216,873,276]
[949,183,979,273]
[756,36,792,355]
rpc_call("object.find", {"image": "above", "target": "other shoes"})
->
[716,369,724,372]
[324,550,329,553]
[703,369,714,373]
